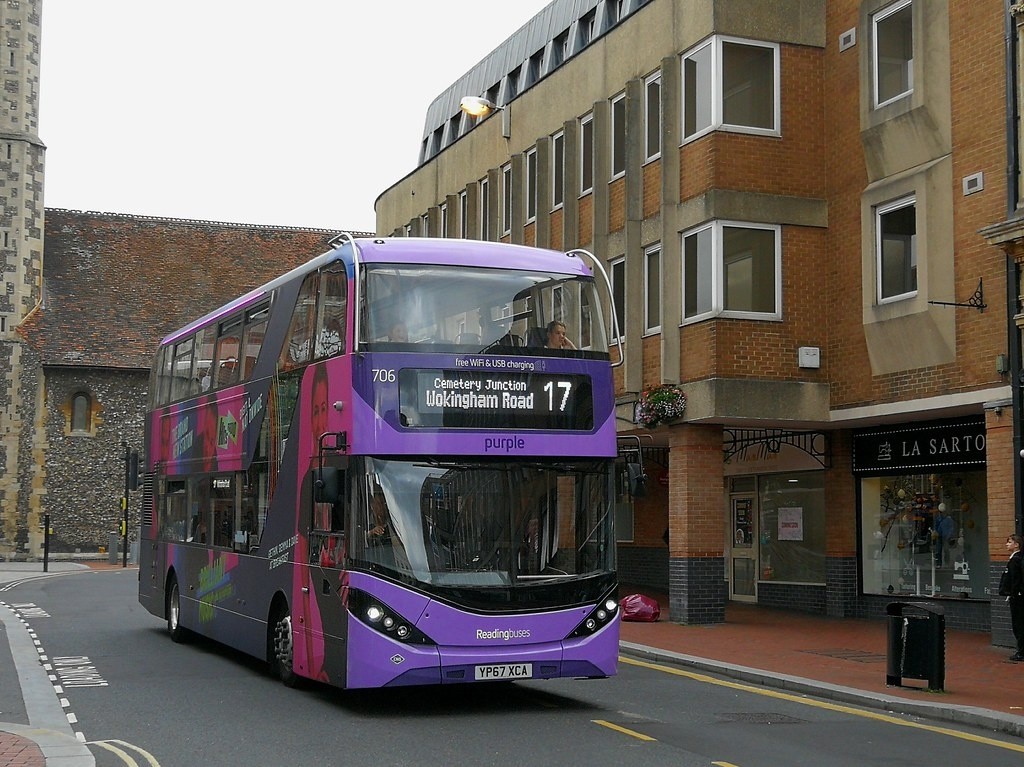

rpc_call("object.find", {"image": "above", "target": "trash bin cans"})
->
[885,601,946,692]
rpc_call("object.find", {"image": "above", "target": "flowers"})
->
[633,385,687,427]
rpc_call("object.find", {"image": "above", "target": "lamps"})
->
[459,96,511,138]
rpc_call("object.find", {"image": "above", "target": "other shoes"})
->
[1008,654,1024,661]
[936,565,941,569]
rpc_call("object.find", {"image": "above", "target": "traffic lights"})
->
[128,448,145,491]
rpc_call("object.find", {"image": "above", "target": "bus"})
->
[136,231,648,692]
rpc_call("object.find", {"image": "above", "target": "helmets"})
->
[938,503,946,511]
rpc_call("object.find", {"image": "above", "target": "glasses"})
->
[938,509,943,514]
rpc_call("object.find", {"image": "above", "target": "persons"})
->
[387,321,410,343]
[542,320,577,351]
[997,533,1024,662]
[361,491,396,539]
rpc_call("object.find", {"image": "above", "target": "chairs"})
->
[170,356,277,392]
[527,326,569,355]
